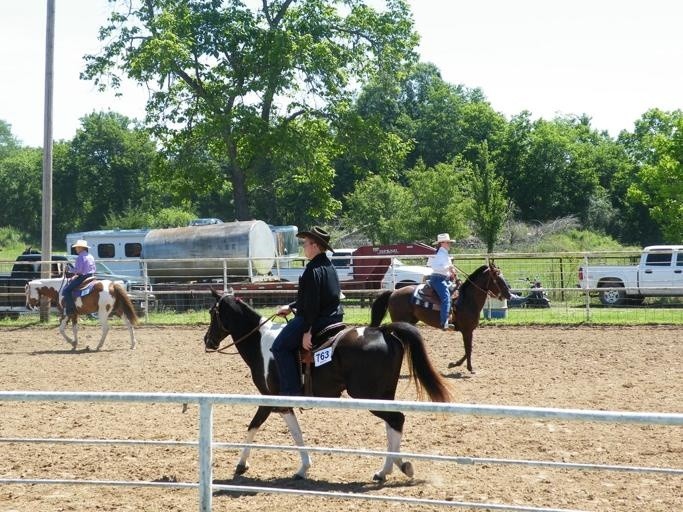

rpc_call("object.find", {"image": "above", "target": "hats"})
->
[295,227,333,254]
[71,240,89,248]
[432,233,453,246]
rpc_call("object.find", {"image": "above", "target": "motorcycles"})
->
[507,278,551,309]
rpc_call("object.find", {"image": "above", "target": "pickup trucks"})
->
[578,245,683,306]
[273,246,435,289]
[0,254,154,321]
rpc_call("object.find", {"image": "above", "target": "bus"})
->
[66,227,444,309]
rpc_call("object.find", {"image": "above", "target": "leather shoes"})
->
[66,311,77,315]
[443,322,455,330]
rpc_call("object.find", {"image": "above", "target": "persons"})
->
[63,240,96,314]
[277,225,344,403]
[429,233,455,332]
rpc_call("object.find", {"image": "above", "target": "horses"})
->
[23,277,142,353]
[201,284,457,482]
[371,258,512,375]
